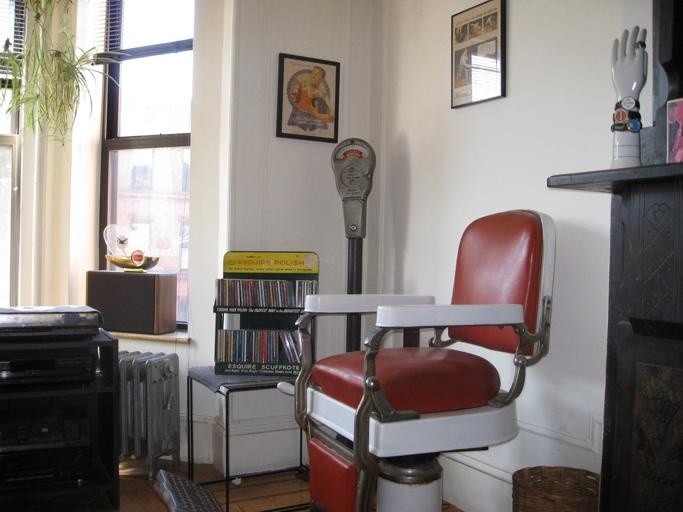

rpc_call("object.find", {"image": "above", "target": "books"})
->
[214,276,313,366]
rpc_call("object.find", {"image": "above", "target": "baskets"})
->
[510,464,600,511]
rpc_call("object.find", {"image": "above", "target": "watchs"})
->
[610,121,642,135]
[612,113,643,122]
[614,96,640,110]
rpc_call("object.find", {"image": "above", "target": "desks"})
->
[187,366,303,512]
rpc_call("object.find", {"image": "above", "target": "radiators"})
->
[118,350,180,479]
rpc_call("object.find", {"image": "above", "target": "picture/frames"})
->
[276,52,340,144]
[451,0,506,108]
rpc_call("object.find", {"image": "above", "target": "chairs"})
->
[295,209,553,512]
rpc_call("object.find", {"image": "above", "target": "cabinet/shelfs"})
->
[0,329,121,512]
[547,1,683,512]
[213,249,319,374]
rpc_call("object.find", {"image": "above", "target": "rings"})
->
[635,41,645,50]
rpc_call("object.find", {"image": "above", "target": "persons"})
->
[667,101,681,164]
[610,26,648,171]
[454,48,473,85]
[289,65,331,125]
